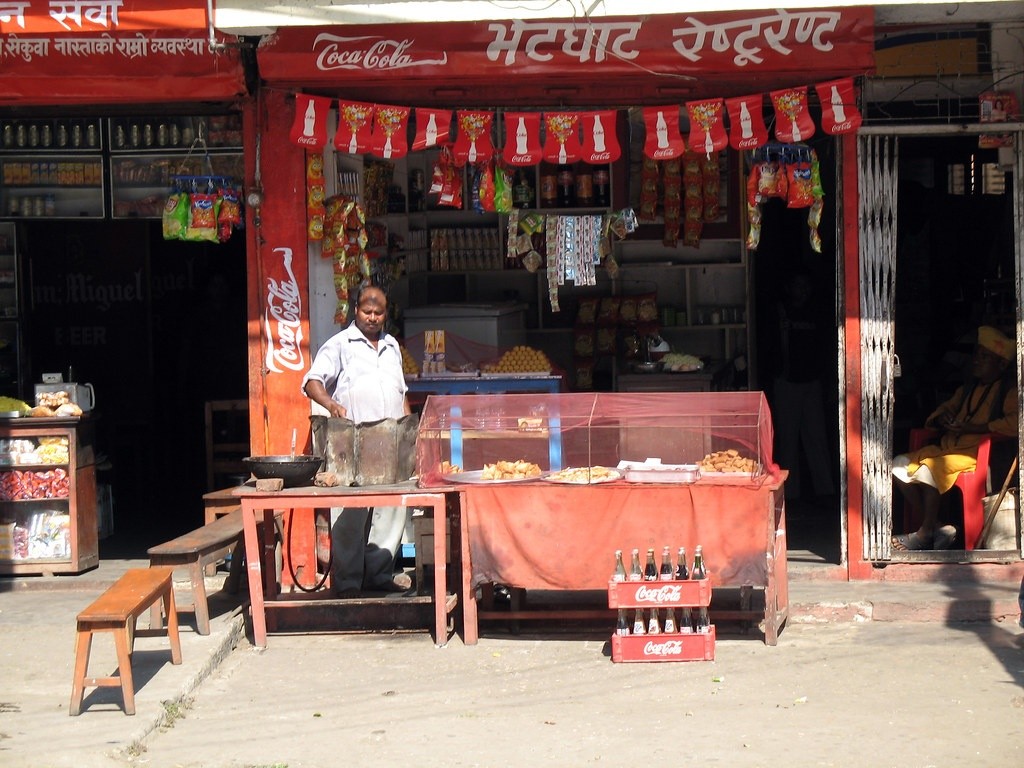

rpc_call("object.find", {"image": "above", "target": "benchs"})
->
[147,510,287,638]
[71,569,183,715]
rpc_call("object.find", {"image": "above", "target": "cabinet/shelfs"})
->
[0,412,100,578]
[0,222,27,399]
[304,104,758,472]
[0,117,244,221]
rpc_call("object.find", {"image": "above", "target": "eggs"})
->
[670,364,698,371]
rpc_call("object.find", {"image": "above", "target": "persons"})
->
[761,269,838,510]
[300,286,410,599]
[891,326,1018,551]
[181,267,243,491]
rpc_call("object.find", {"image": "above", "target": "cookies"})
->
[552,466,620,481]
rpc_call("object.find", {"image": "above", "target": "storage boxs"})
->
[611,623,716,664]
[608,570,714,610]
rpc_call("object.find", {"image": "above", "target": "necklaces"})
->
[954,381,995,447]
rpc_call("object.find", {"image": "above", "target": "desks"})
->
[203,486,241,575]
[231,484,455,648]
[453,469,790,646]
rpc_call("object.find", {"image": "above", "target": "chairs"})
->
[903,429,1013,549]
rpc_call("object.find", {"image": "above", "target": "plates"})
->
[663,370,702,373]
[442,470,549,483]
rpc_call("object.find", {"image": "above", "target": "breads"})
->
[26,391,83,416]
[698,449,758,475]
[399,345,419,374]
[482,346,552,374]
[482,458,542,481]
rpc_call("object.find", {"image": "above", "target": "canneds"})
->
[114,123,182,148]
[3,121,98,147]
[6,193,57,218]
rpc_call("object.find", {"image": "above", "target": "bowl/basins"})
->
[630,361,658,374]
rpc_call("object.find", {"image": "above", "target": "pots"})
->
[242,455,325,485]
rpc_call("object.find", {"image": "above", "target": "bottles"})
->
[613,545,705,583]
[593,165,610,206]
[475,392,508,430]
[616,607,710,634]
[558,165,575,206]
